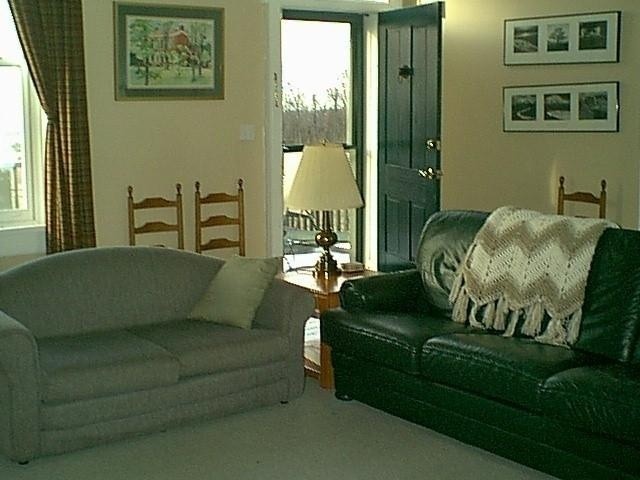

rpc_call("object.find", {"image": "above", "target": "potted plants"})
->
[112,0,225,100]
[501,9,621,133]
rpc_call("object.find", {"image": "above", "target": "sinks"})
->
[1,245,315,465]
[321,213,640,479]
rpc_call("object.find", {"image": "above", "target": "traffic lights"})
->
[187,254,282,331]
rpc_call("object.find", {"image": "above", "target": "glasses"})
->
[285,142,365,278]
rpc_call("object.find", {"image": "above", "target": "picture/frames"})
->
[275,267,391,389]
